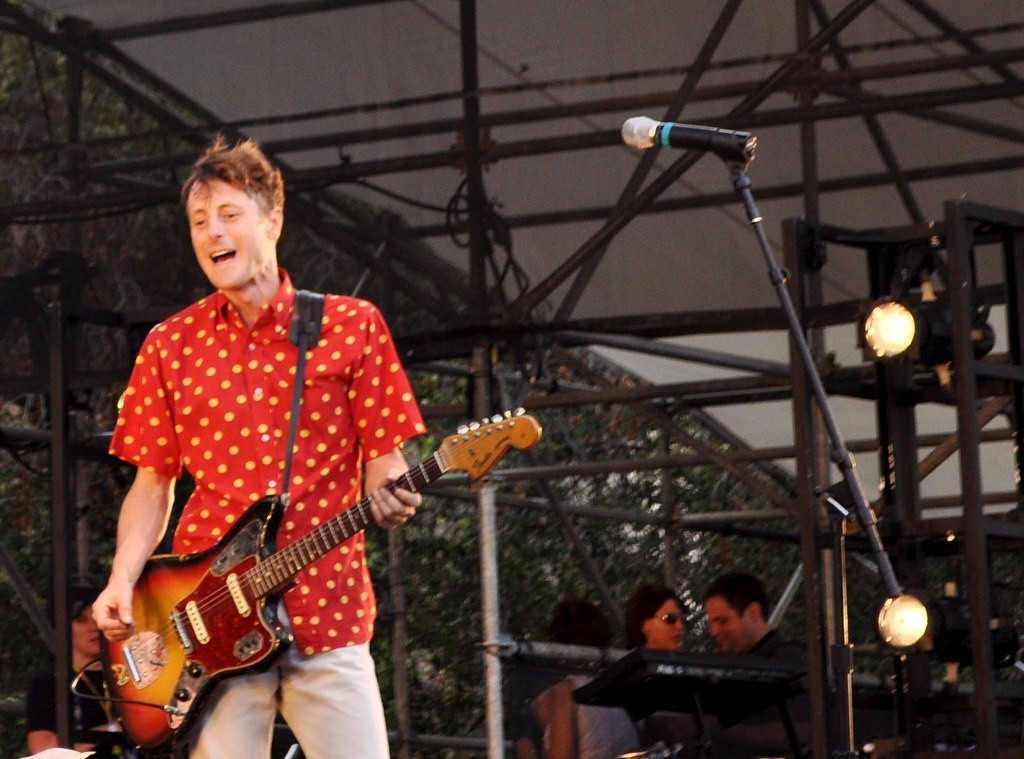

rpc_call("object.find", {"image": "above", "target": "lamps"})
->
[860,294,996,369]
[877,588,1018,670]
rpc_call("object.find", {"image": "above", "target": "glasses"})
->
[661,613,689,625]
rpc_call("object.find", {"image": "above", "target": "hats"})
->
[48,587,104,629]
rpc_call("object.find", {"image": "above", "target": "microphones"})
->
[622,116,756,158]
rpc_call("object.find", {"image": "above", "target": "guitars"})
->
[98,406,543,755]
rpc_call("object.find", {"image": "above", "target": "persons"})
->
[26,587,171,759]
[91,135,426,759]
[517,573,785,759]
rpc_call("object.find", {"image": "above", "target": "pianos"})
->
[567,645,982,759]
